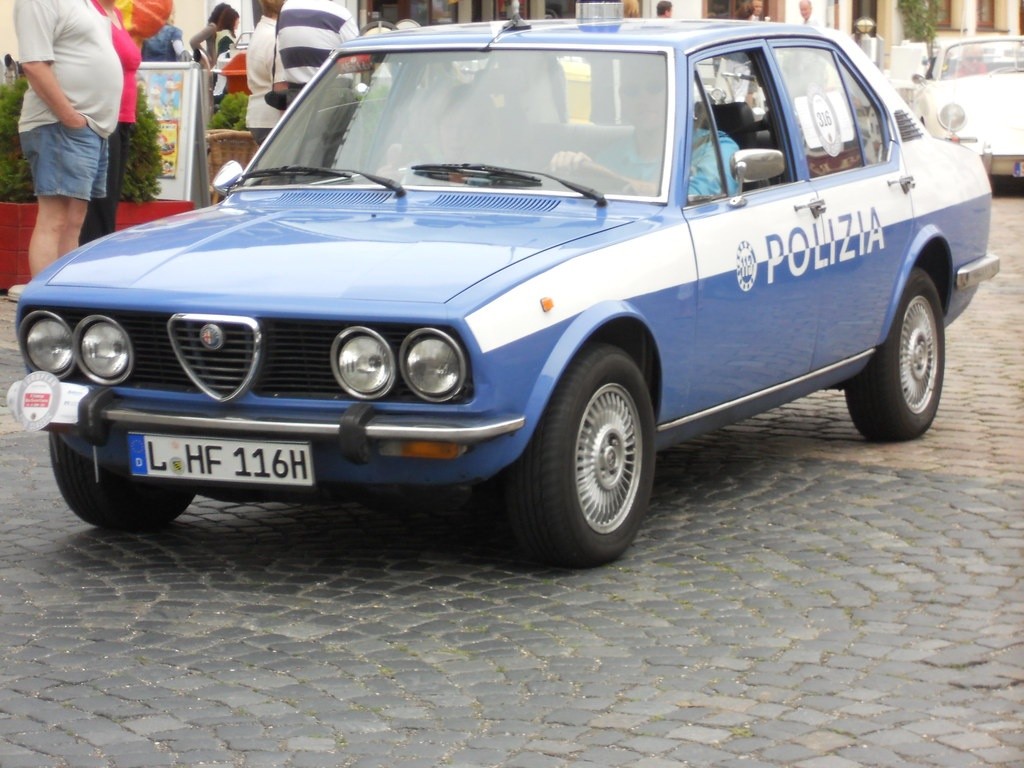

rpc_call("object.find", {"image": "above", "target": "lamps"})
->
[854,16,877,33]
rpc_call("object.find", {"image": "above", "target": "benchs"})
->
[556,114,862,186]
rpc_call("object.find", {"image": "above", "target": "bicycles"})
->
[710,60,755,105]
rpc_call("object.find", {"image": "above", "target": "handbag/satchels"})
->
[264,90,287,110]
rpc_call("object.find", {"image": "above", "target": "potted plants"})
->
[0,74,197,294]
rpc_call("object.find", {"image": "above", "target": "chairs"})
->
[205,128,259,204]
[709,103,766,191]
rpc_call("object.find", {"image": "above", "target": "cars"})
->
[5,3,1001,568]
[912,36,1024,179]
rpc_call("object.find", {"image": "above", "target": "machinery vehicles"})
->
[193,19,423,131]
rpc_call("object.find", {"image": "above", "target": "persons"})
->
[79,0,142,248]
[277,0,360,109]
[799,0,825,27]
[622,0,639,18]
[749,0,770,21]
[14,0,124,280]
[190,4,231,68]
[657,1,673,19]
[549,67,743,197]
[737,2,753,20]
[213,8,240,60]
[141,14,192,62]
[246,0,285,147]
